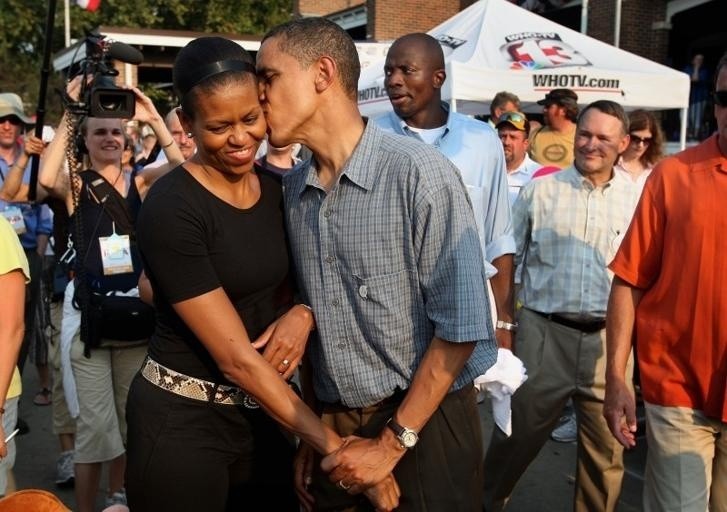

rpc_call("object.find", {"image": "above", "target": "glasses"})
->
[499,112,522,122]
[710,89,727,108]
[630,134,654,145]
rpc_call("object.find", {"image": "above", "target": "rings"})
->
[339,479,350,490]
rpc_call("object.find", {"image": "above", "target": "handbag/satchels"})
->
[73,239,154,345]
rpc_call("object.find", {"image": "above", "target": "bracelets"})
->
[496,320,518,332]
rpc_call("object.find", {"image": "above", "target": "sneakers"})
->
[33,383,53,404]
[104,487,127,508]
[55,450,76,488]
[551,413,578,442]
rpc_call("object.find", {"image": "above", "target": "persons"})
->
[0,91,304,500]
[368,32,520,360]
[600,56,727,511]
[549,107,666,444]
[484,87,581,322]
[475,99,644,511]
[37,70,188,512]
[683,52,710,142]
[254,16,497,510]
[124,35,402,512]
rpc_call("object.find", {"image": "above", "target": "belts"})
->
[139,353,297,409]
[527,308,607,335]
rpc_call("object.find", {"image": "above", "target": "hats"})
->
[537,94,577,105]
[494,111,531,131]
[142,123,156,139]
[0,92,36,135]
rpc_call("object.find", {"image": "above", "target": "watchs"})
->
[384,416,421,451]
